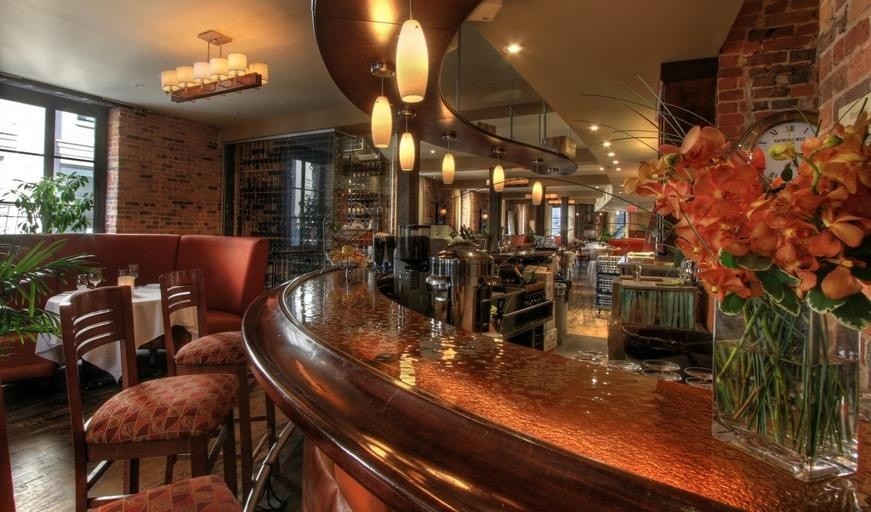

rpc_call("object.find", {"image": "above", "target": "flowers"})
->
[447,72,870,461]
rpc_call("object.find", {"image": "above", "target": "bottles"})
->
[235,133,387,289]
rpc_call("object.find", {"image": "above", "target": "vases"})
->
[710,294,862,484]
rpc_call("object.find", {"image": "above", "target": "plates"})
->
[570,348,714,391]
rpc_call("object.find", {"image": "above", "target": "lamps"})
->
[367,0,430,174]
[491,148,506,193]
[158,29,269,104]
[531,158,544,206]
[441,130,456,186]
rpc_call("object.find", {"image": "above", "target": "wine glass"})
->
[75,264,141,298]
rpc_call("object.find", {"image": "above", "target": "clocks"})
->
[736,109,818,188]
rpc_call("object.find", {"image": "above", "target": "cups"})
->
[631,260,642,283]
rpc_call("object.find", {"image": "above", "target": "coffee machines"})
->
[392,224,431,318]
